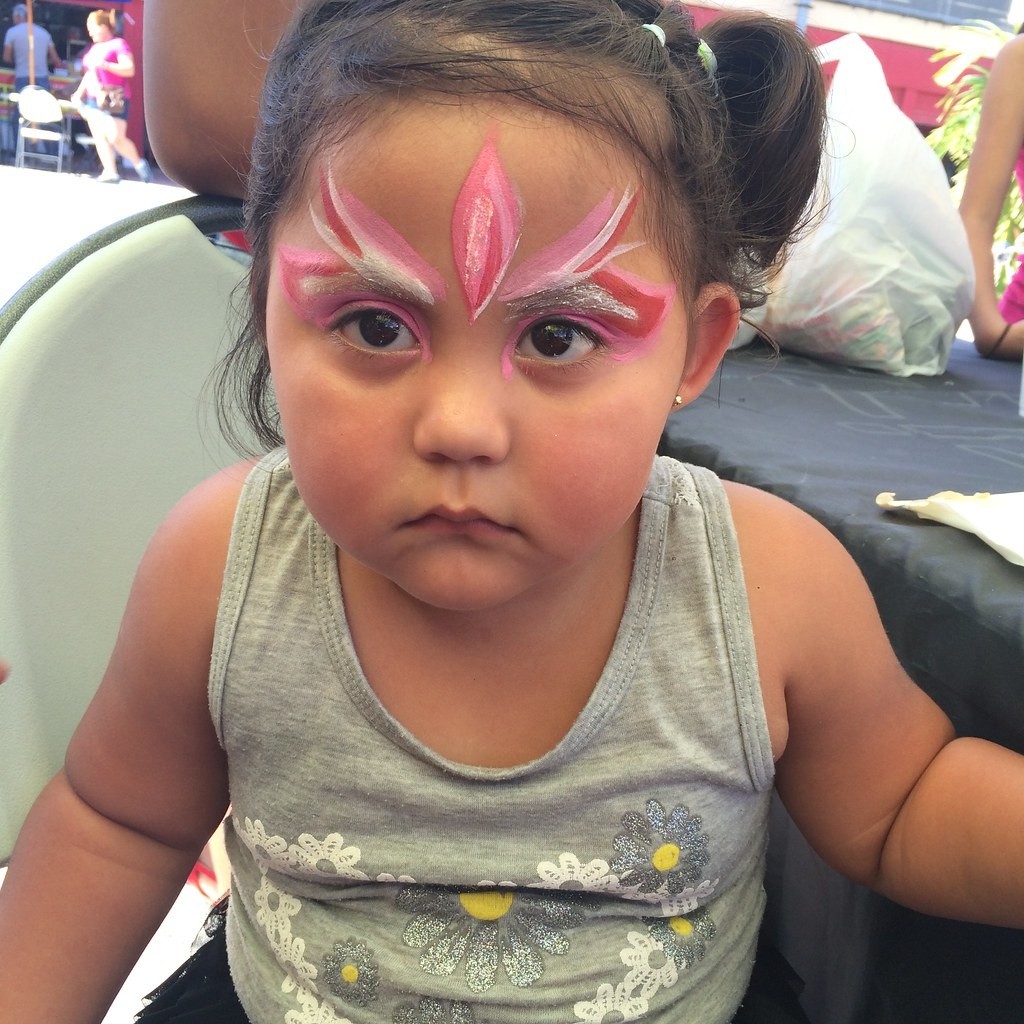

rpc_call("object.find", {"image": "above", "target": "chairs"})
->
[16,84,74,170]
[0,193,292,870]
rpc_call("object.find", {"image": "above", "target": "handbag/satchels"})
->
[96,85,125,114]
[759,31,975,378]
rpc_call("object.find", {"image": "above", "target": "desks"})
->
[644,315,1024,1024]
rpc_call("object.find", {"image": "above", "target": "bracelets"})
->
[989,324,1011,356]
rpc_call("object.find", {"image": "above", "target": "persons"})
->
[71,10,154,182]
[961,33,1024,360]
[143,0,300,197]
[4,5,62,167]
[0,0,1024,1024]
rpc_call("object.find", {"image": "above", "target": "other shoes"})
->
[136,157,153,183]
[97,174,120,184]
